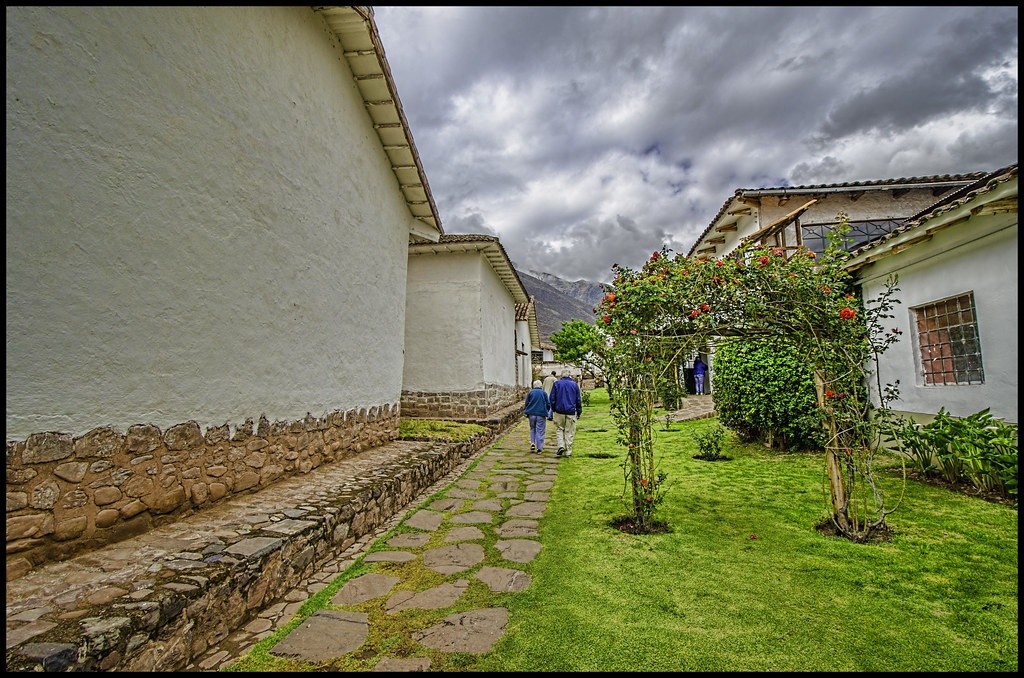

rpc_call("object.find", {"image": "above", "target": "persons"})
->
[542,370,557,420]
[549,369,582,458]
[524,379,551,454]
[693,356,708,395]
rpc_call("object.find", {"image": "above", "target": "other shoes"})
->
[536,449,543,454]
[557,446,564,455]
[567,455,571,457]
[696,393,698,396]
[700,392,704,396]
[530,444,535,450]
